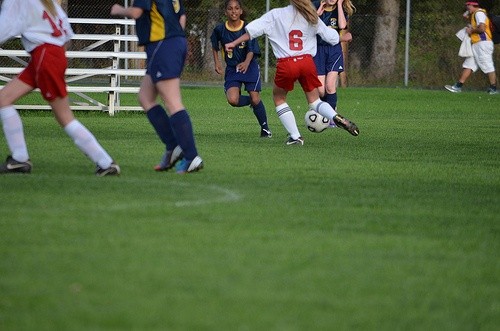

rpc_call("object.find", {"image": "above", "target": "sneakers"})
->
[286,136,304,146]
[333,114,359,136]
[0,154,33,174]
[444,84,462,94]
[488,88,498,94]
[94,161,121,176]
[176,156,203,172]
[154,144,183,171]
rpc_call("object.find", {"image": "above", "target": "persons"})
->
[310,0,356,128]
[188,21,206,75]
[443,0,499,95]
[0,0,120,178]
[224,0,359,144]
[110,0,205,173]
[210,0,273,138]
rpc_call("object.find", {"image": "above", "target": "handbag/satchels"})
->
[457,32,473,58]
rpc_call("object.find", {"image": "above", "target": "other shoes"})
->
[260,129,272,138]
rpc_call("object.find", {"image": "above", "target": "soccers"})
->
[305,110,330,133]
